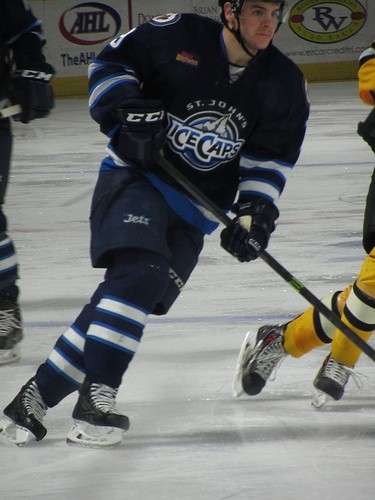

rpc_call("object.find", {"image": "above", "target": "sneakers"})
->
[0,376,48,448]
[67,379,129,448]
[234,323,288,399]
[0,305,24,365]
[311,353,369,410]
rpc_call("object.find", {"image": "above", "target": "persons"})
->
[232,41,375,410]
[0,0,56,364]
[0,0,307,449]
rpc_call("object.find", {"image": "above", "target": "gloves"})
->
[220,197,280,263]
[5,63,54,124]
[110,98,169,167]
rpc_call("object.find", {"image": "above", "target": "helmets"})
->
[218,0,285,56]
